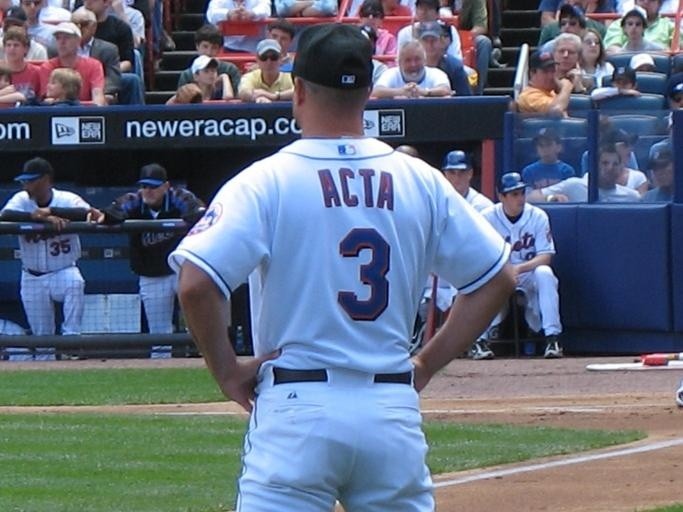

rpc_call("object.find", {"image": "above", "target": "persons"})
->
[0,159,102,364]
[500,0,680,207]
[420,151,494,358]
[167,21,516,511]
[0,320,35,362]
[470,173,562,358]
[85,164,207,358]
[167,1,502,105]
[1,1,175,104]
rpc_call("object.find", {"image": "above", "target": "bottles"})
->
[235,326,246,354]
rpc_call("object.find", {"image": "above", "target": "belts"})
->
[273,368,411,385]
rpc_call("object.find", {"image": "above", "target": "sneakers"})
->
[544,334,563,358]
[466,339,495,361]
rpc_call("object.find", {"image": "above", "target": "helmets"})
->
[441,149,472,169]
[498,172,527,193]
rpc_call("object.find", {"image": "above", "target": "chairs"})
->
[514,53,683,202]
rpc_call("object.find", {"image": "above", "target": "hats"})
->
[136,163,169,186]
[528,5,682,168]
[14,158,54,182]
[288,23,374,88]
